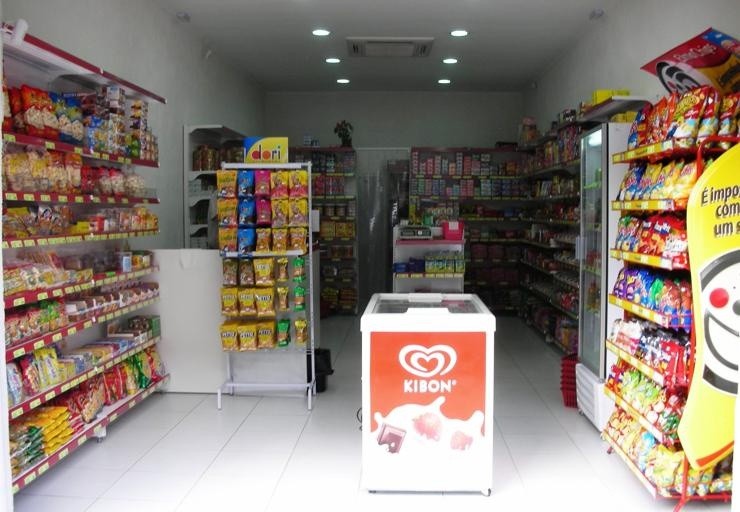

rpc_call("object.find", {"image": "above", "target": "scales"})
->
[400,226,433,240]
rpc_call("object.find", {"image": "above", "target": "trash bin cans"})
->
[307,348,333,392]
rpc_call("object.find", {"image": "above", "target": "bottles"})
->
[587,279,597,306]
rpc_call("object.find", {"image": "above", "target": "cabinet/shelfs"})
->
[392,218,465,294]
[385,154,407,292]
[182,120,254,250]
[523,113,587,361]
[217,160,320,397]
[406,141,533,318]
[600,118,739,510]
[1,24,168,492]
[293,146,363,319]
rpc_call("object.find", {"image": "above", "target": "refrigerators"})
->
[574,118,637,433]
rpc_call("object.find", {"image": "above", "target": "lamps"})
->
[526,78,541,91]
[1,17,31,49]
[201,45,214,62]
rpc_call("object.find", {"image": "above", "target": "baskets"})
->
[560,353,578,407]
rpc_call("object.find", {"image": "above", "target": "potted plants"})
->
[334,118,355,149]
